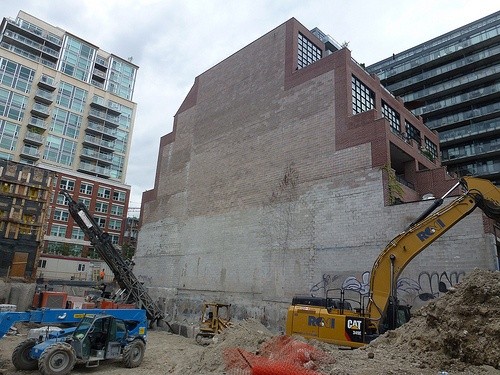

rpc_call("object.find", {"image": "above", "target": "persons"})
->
[99,269,105,281]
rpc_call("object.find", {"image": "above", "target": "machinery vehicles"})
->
[286,174,500,348]
[194,301,237,346]
[0,306,148,375]
[22,188,163,332]
